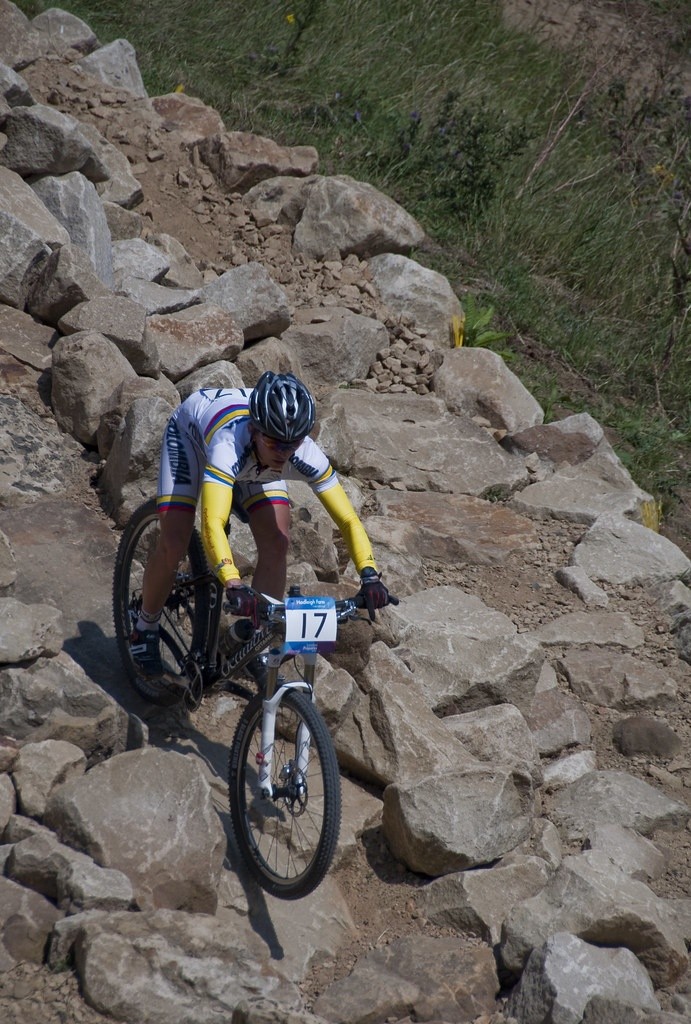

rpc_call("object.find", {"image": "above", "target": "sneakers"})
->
[248,653,285,688]
[128,624,163,677]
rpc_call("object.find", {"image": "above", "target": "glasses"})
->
[254,428,305,452]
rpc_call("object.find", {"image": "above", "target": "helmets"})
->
[248,372,316,443]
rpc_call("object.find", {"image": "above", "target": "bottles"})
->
[218,619,255,657]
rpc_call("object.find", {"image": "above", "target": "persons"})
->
[130,371,389,678]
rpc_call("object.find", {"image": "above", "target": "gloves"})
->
[226,586,261,629]
[355,572,389,621]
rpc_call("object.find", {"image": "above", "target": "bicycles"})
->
[110,497,400,901]
